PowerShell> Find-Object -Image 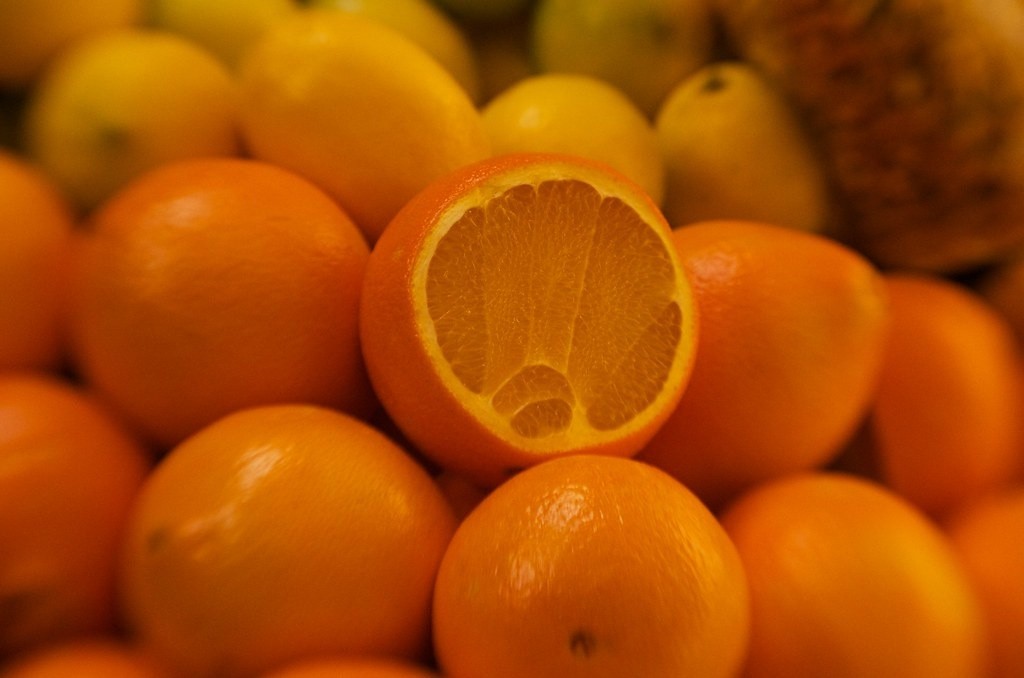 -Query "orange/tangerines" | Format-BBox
[1,0,1024,678]
